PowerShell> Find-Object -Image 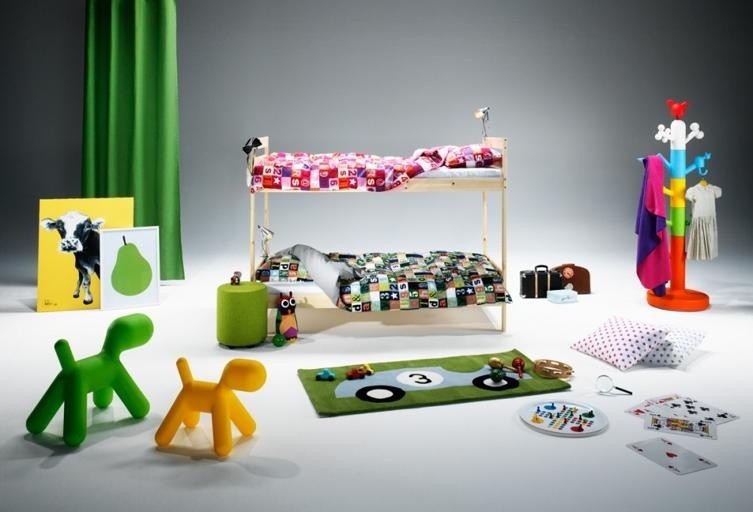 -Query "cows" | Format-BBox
[39,211,104,304]
